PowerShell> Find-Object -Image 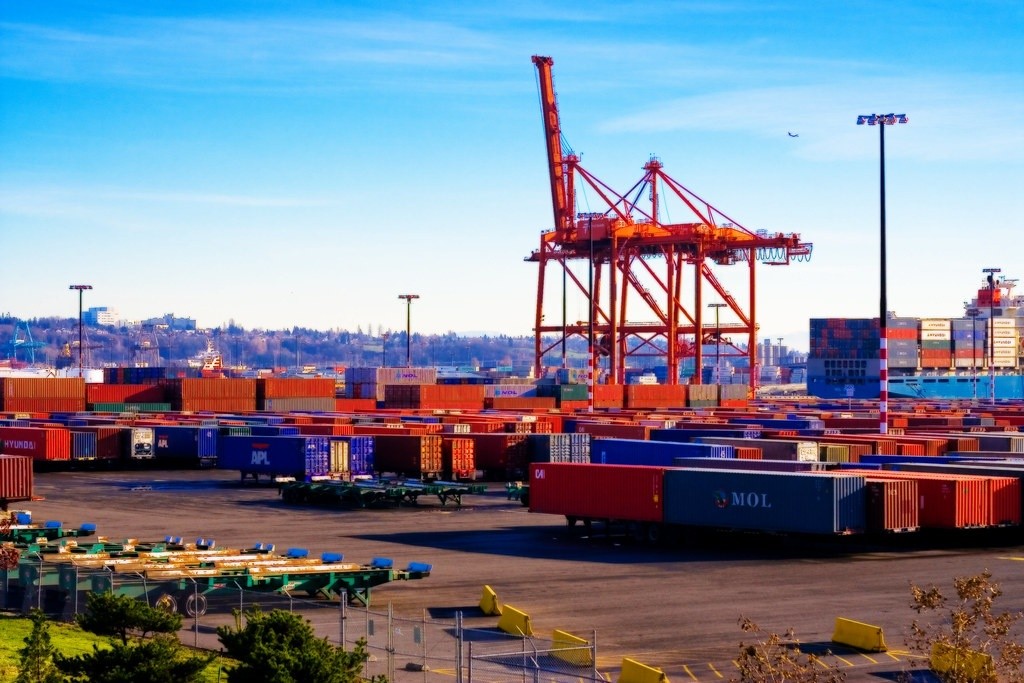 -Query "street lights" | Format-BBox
[857,113,909,435]
[399,295,420,368]
[983,268,1002,405]
[70,285,93,378]
